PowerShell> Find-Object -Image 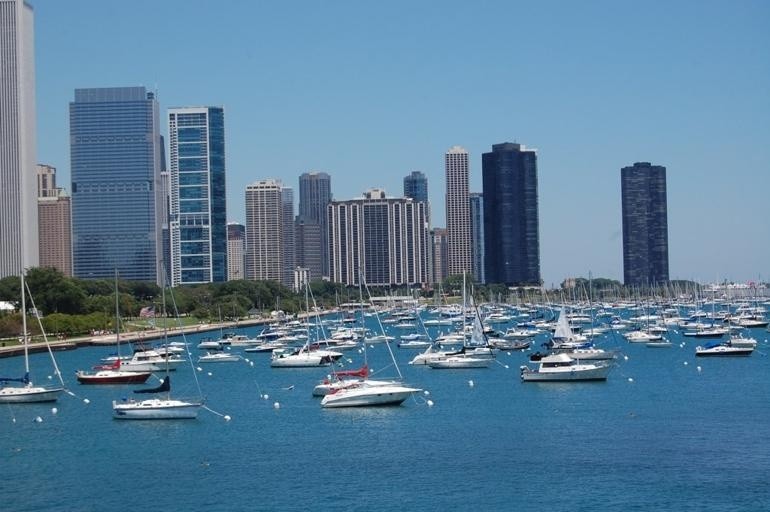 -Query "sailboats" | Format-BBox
[198,276,342,367]
[75,263,202,419]
[1,274,64,403]
[313,268,422,407]
